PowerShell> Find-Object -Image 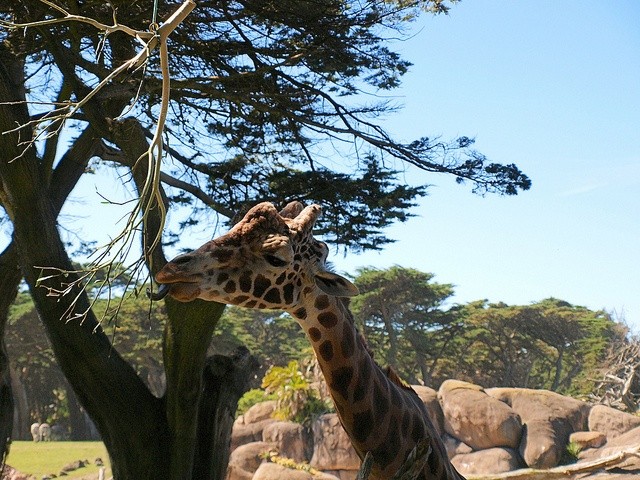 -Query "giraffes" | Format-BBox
[153,198,467,480]
